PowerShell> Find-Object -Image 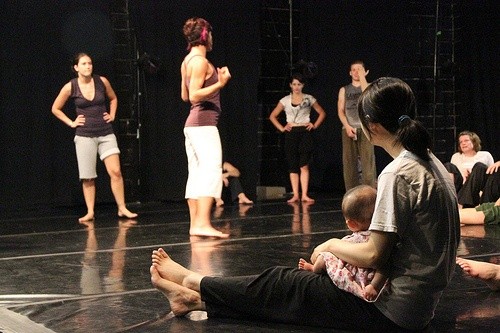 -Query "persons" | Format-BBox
[337,58,377,192]
[51,51,138,222]
[442,131,500,226]
[180,17,232,238]
[298,185,391,303]
[269,74,327,202]
[149,77,461,333]
[215,161,254,207]
[455,256,500,291]
